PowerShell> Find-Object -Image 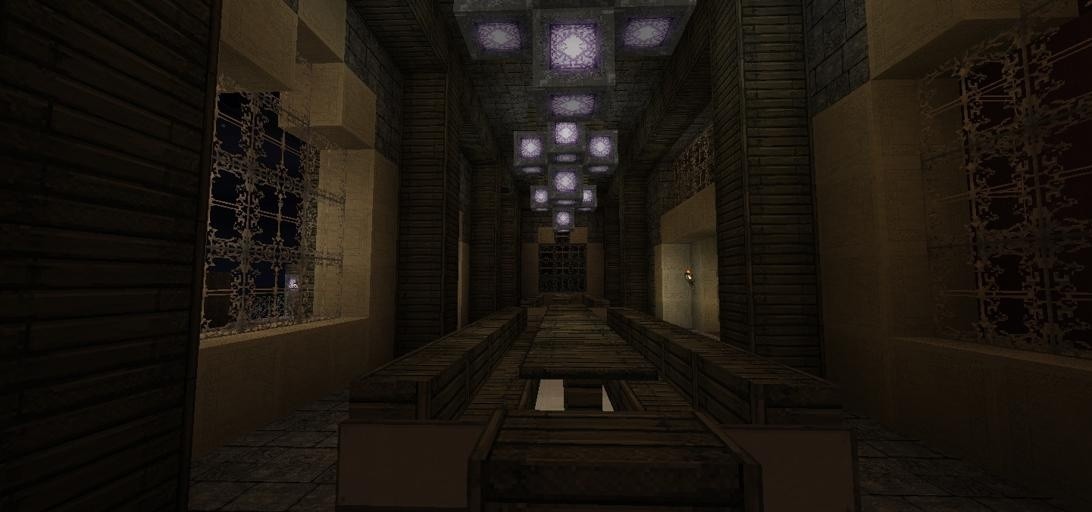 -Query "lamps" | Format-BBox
[450,1,701,237]
